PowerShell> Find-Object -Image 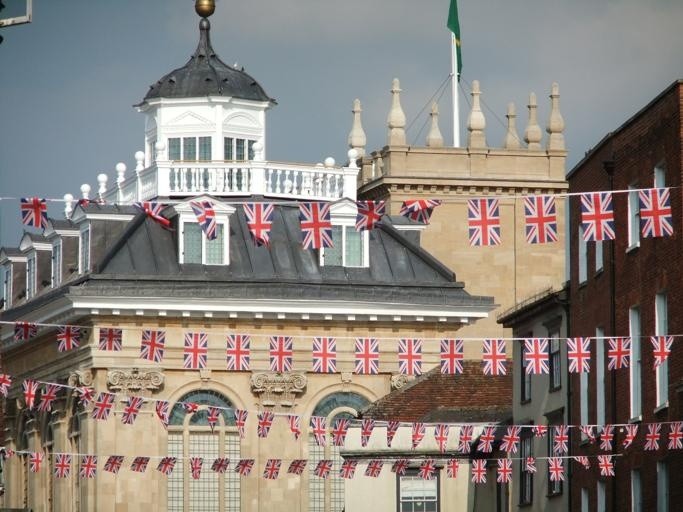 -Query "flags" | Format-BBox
[299,202,333,250]
[76,197,101,210]
[580,192,617,241]
[639,187,673,238]
[242,201,273,247]
[20,196,48,227]
[467,199,502,247]
[447,0,463,85]
[399,199,441,225]
[191,201,219,241]
[134,202,175,233]
[0,323,682,489]
[524,196,558,244]
[355,201,386,230]
